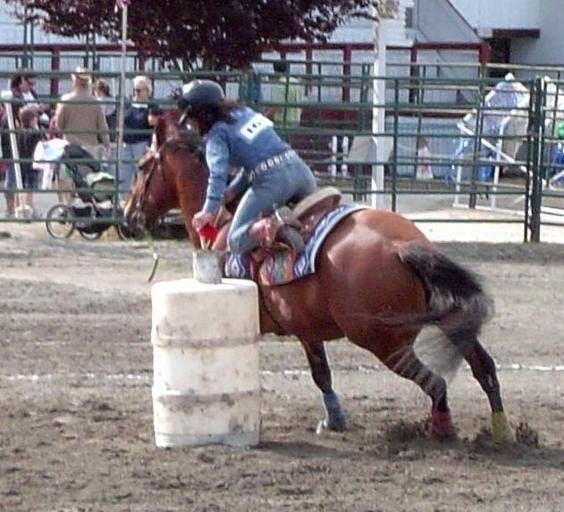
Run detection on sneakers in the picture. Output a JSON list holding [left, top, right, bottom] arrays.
[[59, 212, 67, 224]]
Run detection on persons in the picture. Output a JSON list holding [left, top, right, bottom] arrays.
[[172, 78, 321, 258], [0, 66, 167, 226]]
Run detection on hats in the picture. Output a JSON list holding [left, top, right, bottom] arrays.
[[72, 67, 91, 81], [133, 76, 149, 87]]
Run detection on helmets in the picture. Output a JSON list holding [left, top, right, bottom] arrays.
[[178, 79, 225, 127]]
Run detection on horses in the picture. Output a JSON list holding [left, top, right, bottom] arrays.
[[120, 101, 514, 444]]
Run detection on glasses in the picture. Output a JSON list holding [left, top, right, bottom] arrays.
[[25, 79, 37, 85]]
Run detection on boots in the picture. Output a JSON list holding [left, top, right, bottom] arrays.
[[252, 206, 305, 253]]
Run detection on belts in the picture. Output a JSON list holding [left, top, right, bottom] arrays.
[[247, 149, 294, 181]]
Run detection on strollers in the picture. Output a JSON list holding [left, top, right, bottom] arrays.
[[45, 144, 146, 243]]
